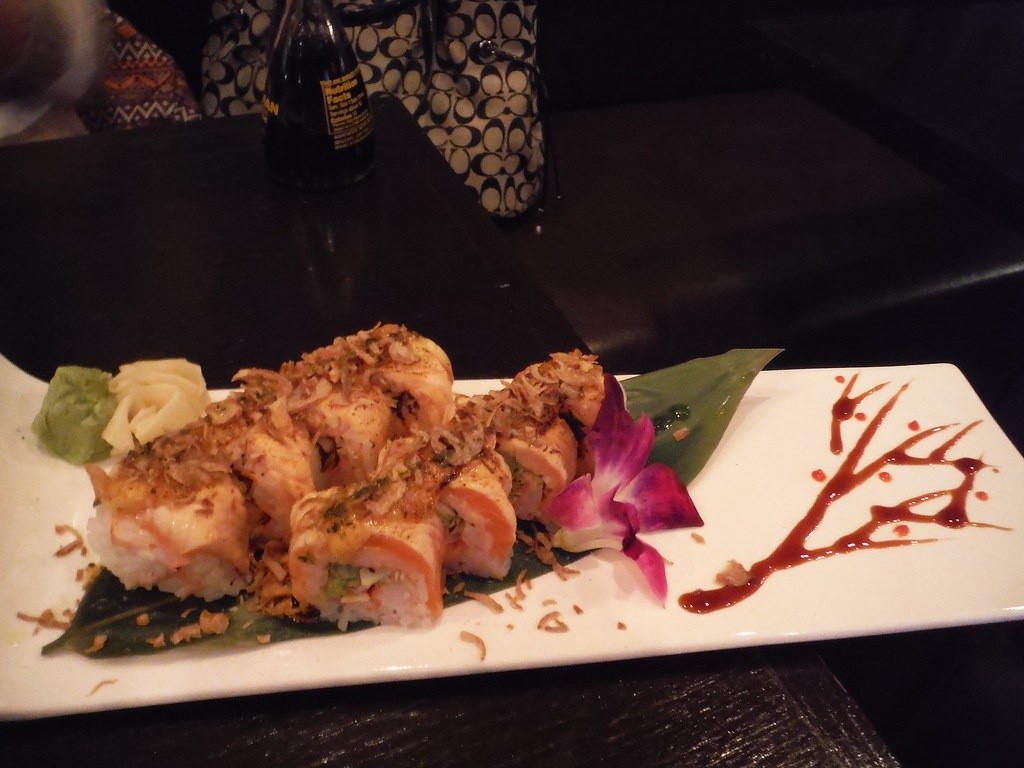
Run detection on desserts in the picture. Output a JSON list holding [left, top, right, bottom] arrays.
[[88, 323, 605, 630]]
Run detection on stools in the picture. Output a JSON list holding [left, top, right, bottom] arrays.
[[497, 0, 1023, 370]]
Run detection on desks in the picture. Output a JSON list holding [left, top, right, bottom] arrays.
[[745, 9, 1023, 202]]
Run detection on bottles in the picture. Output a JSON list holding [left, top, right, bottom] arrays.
[[265, 0, 378, 189]]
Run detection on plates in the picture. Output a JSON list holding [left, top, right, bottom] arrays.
[[0, 351, 1024, 721]]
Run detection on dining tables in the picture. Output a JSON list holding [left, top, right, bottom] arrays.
[[0, 90, 906, 768]]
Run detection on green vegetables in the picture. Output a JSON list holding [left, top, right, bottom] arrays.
[[31, 364, 110, 465]]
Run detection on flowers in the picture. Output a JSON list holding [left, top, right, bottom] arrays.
[[545, 368, 705, 611]]
